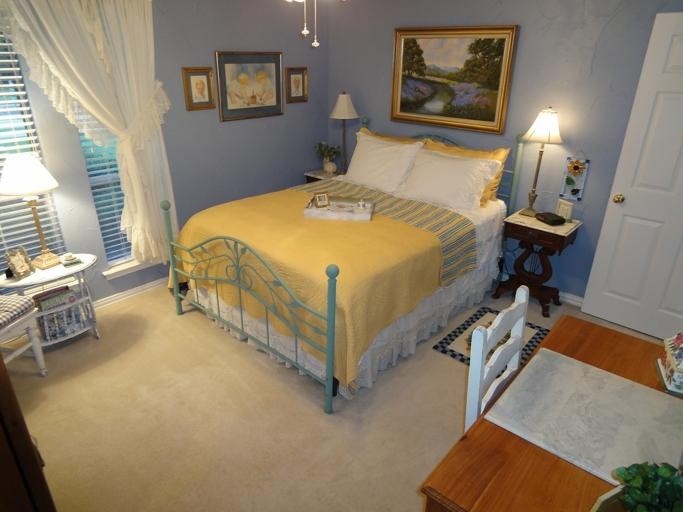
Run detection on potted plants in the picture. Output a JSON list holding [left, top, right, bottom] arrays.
[[592, 461, 683, 510], [314, 140, 345, 175]]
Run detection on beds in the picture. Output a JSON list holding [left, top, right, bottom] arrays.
[[157, 115, 526, 414]]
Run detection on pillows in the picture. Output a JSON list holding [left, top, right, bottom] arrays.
[[337, 129, 421, 199], [396, 148, 501, 217]]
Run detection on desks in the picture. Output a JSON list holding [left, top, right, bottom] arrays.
[[0, 251, 99, 350], [417, 315, 683, 511], [492, 207, 582, 317]]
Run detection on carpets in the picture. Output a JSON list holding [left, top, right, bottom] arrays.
[[431, 306, 551, 379]]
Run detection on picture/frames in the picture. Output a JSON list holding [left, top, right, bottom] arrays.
[[213, 51, 286, 122], [181, 66, 215, 112], [286, 67, 307, 106], [391, 23, 518, 134]]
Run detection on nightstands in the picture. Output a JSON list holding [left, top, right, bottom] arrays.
[[303, 167, 334, 181]]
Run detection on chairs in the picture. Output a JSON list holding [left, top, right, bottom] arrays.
[[0, 287, 50, 381], [464, 284, 531, 430]]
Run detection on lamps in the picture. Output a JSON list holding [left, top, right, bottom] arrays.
[[0, 149, 69, 269], [518, 103, 562, 217], [326, 93, 360, 174], [282, 1, 328, 48]]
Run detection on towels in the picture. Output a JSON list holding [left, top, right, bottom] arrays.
[[486, 347, 683, 489]]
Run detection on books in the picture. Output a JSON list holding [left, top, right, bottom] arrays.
[[30, 285, 81, 339]]
